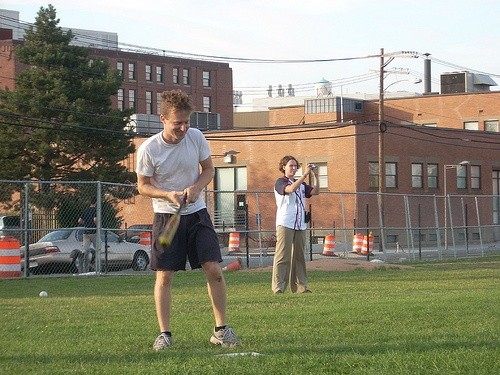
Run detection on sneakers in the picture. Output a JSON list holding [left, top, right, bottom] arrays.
[[152, 331, 173, 352], [90, 263, 96, 271]]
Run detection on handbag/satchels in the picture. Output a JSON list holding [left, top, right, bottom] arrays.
[[210, 325, 242, 349]]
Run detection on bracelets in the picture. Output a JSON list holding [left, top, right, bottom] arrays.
[[302, 174, 307, 178]]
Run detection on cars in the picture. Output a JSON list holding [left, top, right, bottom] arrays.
[[119, 224, 152, 240], [0, 215, 21, 237], [19, 226, 151, 275]]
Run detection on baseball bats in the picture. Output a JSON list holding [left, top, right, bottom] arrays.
[[157, 193, 187, 247]]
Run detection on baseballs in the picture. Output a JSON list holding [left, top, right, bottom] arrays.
[[39, 291, 48, 297]]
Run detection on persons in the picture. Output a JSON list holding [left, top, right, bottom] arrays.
[[135, 88, 244, 351], [77, 197, 98, 273], [271, 155, 320, 294]]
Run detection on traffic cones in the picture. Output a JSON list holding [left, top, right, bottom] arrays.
[[221, 257, 244, 272]]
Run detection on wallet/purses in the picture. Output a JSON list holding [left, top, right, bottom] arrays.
[[305, 211, 312, 224]]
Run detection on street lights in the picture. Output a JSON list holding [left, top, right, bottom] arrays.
[[444, 161, 472, 250], [378, 78, 423, 253], [204, 150, 241, 209]]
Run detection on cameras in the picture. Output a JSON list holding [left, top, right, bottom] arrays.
[[309, 163, 317, 170]]
